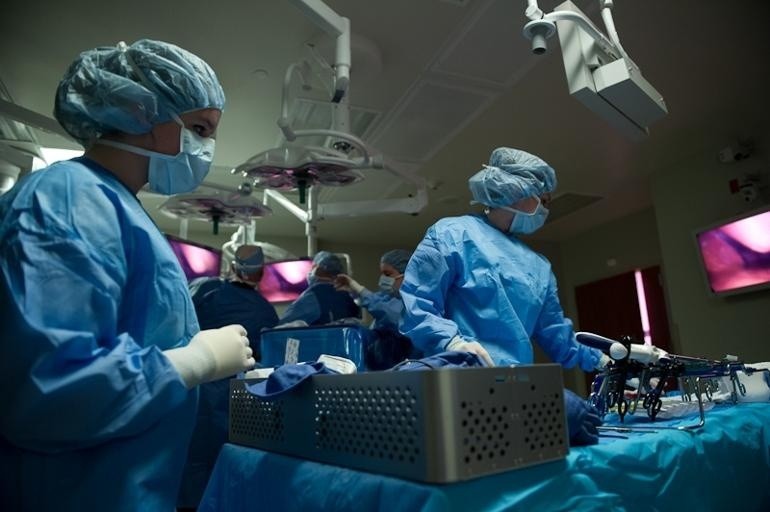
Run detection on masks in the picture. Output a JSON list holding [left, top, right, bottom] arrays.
[[494, 195, 550, 236], [96, 114, 219, 195], [378, 274, 405, 290]]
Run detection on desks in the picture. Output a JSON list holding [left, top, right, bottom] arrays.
[[217, 391, 770, 511]]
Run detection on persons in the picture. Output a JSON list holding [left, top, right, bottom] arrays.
[[333, 249, 415, 367], [397, 145, 613, 372], [191, 245, 278, 459], [276, 251, 360, 326], [0, 39, 258, 512]]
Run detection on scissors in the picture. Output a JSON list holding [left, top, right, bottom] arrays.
[[588, 370, 747, 424]]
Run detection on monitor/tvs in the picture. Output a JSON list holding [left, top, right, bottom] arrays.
[[161, 231, 223, 282], [690, 203, 770, 299], [257, 256, 314, 303]]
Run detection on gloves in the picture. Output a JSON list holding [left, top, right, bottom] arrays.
[[447, 335, 495, 369], [160, 323, 255, 390], [331, 273, 369, 297]]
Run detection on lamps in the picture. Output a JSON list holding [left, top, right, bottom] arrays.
[[155, 142, 366, 236]]
